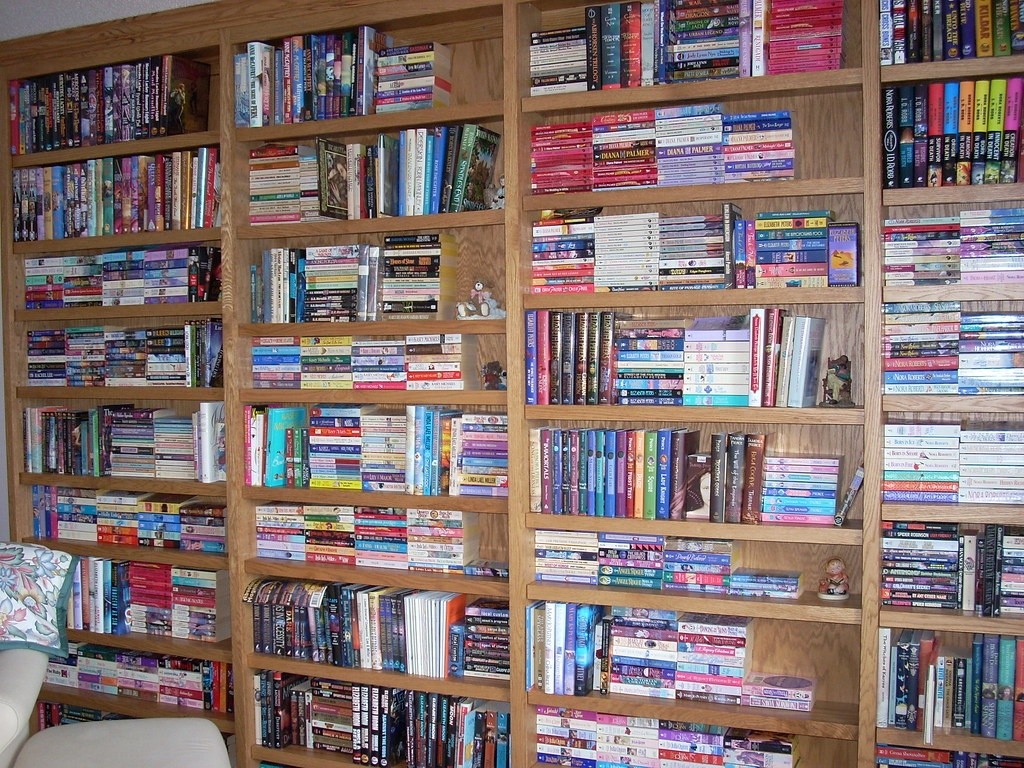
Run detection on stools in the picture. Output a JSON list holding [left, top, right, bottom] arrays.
[[14, 718, 232, 768]]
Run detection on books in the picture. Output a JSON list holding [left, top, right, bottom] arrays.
[[10, 0, 1024, 768]]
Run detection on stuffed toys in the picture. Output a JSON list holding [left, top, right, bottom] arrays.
[[479, 360, 507, 391], [457, 277, 506, 319]]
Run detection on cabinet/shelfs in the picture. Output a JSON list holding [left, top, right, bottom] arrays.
[[0, 0, 1024, 768]]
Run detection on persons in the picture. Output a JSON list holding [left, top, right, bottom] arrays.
[[828, 355, 851, 404], [823, 558, 850, 594]]
